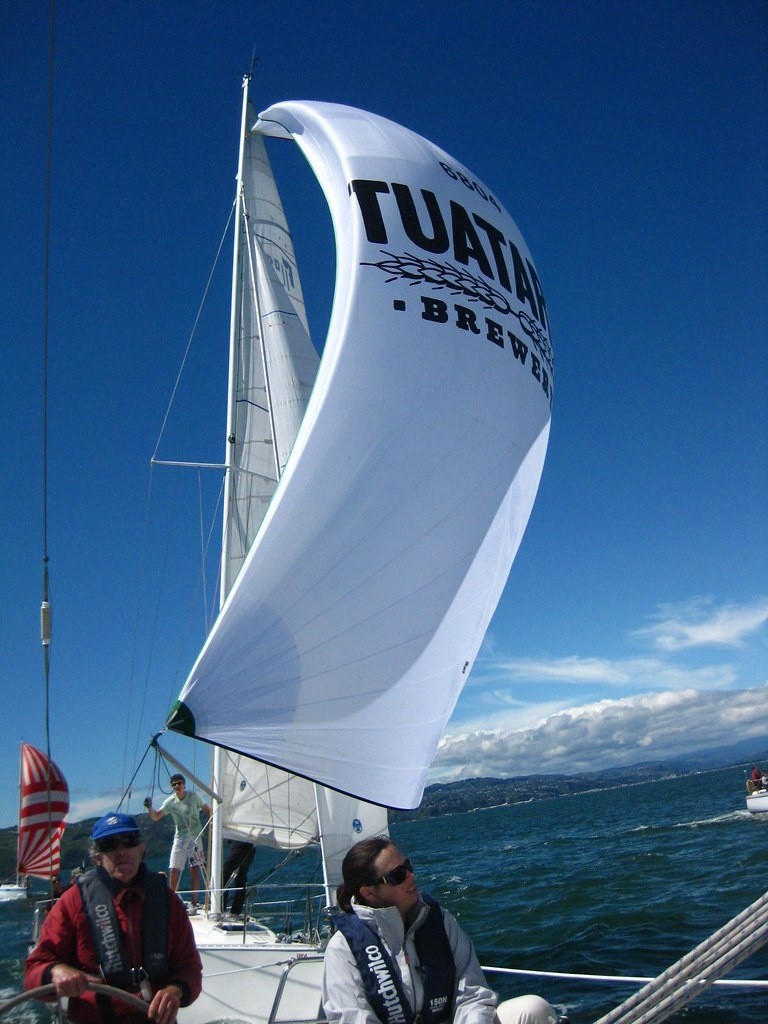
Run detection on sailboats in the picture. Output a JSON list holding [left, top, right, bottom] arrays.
[[1, 731, 72, 904]]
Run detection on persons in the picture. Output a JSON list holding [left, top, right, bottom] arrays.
[[44, 866, 85, 918], [751, 765, 768, 792], [144, 773, 211, 915], [25, 812, 203, 1024], [322, 836, 557, 1024]]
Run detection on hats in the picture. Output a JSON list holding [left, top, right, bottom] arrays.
[[763, 769, 768, 773], [752, 764, 758, 768], [72, 867, 83, 877], [90, 812, 141, 841]]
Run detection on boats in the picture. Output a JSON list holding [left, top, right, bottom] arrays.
[[743, 760, 768, 815]]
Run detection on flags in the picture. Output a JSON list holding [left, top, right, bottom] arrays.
[[19, 741, 70, 882]]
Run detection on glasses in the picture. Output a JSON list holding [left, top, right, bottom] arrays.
[[172, 781, 183, 786], [95, 835, 139, 852], [367, 858, 414, 887], [53, 878, 61, 882]]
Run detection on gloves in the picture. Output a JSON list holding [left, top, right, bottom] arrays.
[[143, 797, 153, 809]]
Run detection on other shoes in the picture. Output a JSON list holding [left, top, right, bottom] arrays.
[[188, 903, 198, 917], [225, 913, 239, 918]]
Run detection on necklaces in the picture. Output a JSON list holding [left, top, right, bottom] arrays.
[[222, 839, 256, 918]]
